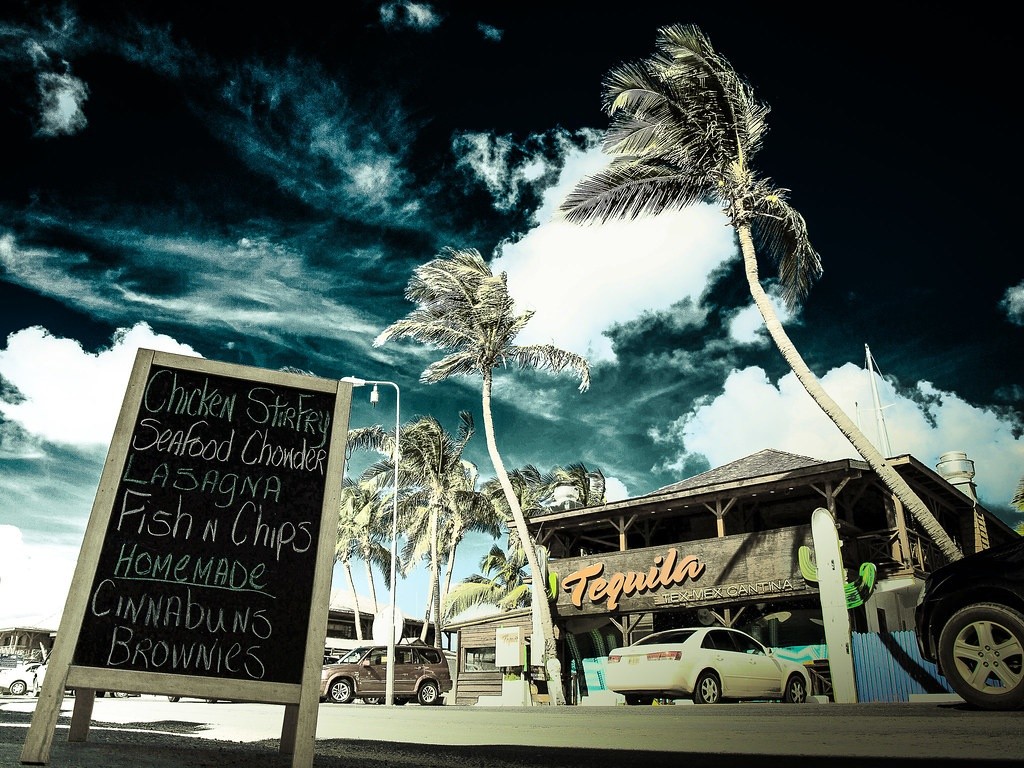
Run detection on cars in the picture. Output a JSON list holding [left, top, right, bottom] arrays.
[[33, 649, 106, 698], [606, 627, 811, 704], [314, 656, 382, 704], [914, 536, 1024, 711], [0, 663, 43, 695]]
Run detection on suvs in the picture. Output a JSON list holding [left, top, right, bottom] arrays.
[[319, 646, 454, 706]]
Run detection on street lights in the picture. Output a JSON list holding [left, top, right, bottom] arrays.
[[338, 375, 405, 707]]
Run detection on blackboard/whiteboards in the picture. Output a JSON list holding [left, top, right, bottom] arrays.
[[64, 347, 353, 705]]
